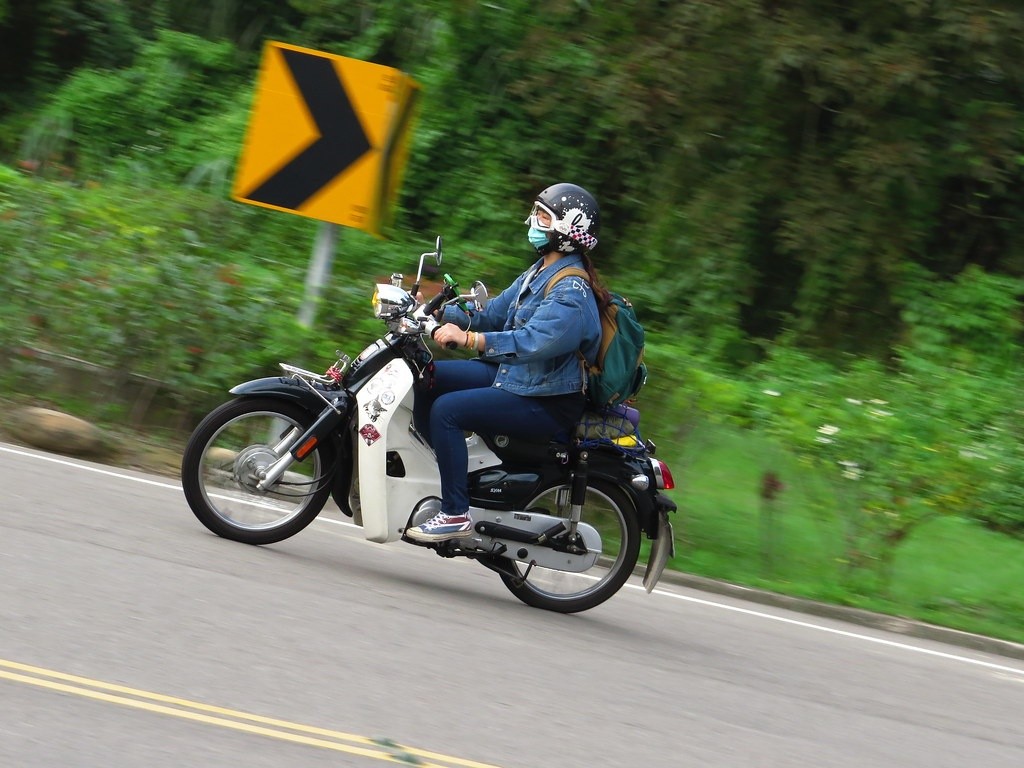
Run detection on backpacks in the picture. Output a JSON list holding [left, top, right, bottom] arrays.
[[544, 266, 648, 414]]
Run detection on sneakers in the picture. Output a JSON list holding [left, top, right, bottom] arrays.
[[406, 511, 475, 544]]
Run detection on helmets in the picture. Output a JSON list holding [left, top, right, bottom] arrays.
[[524, 182, 601, 252]]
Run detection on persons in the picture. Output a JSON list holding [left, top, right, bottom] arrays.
[[406, 183, 603, 543]]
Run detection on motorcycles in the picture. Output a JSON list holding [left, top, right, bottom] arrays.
[[179, 231, 684, 613]]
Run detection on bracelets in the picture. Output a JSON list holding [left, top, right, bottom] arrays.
[[464, 331, 479, 351]]
